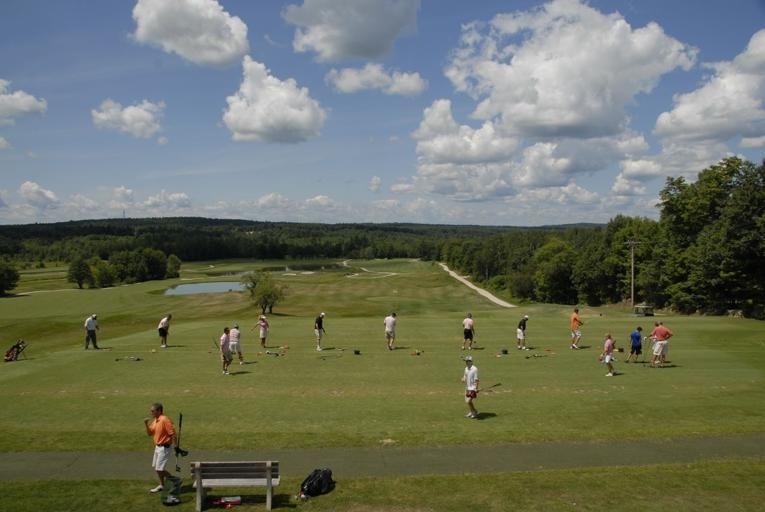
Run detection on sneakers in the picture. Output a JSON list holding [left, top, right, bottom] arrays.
[[467, 412, 472, 417], [472, 411, 477, 419], [605, 373, 613, 377], [151, 485, 162, 492]]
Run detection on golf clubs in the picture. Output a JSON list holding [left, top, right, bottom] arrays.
[[525, 334, 532, 347], [477, 382, 502, 398], [643, 334, 655, 365], [321, 329, 334, 348], [176, 410, 185, 468]]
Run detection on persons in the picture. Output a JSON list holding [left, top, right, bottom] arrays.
[[143, 402, 179, 494], [462, 355, 480, 418], [158, 314, 172, 348], [462, 314, 475, 349], [625, 326, 643, 363], [85, 314, 100, 350], [516, 315, 528, 350], [600, 333, 616, 377], [229, 324, 244, 365], [571, 308, 583, 349], [384, 313, 397, 350], [315, 312, 325, 351], [645, 321, 673, 367], [220, 327, 233, 374], [252, 315, 269, 347]]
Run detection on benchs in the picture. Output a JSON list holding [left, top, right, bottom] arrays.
[[190, 459, 281, 512]]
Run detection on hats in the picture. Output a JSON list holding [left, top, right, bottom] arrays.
[[464, 355, 473, 361]]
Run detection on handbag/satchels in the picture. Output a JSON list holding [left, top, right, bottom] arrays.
[[301, 467, 336, 497]]
